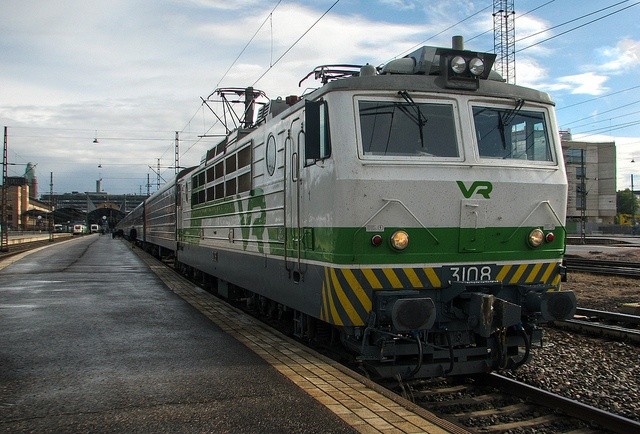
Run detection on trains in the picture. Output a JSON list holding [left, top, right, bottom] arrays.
[[72, 223, 89, 236], [112, 37, 577, 396], [90, 222, 102, 235]]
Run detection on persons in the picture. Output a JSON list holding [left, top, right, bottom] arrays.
[[129, 226, 137, 248]]
